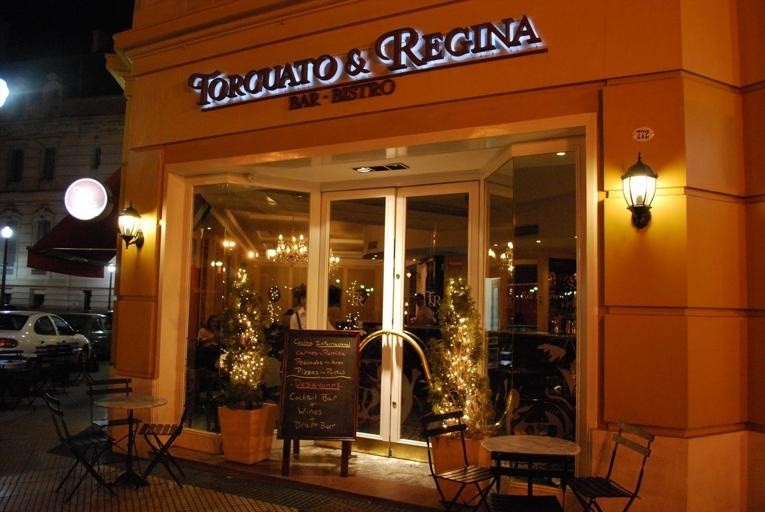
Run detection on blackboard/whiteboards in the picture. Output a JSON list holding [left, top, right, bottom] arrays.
[[277, 329, 361, 441]]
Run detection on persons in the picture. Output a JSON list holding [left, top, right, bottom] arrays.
[[287, 289, 339, 332], [409, 293, 437, 326], [197, 314, 222, 414], [282, 308, 295, 328]]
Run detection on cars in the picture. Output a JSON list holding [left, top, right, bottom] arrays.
[[1, 309, 112, 373]]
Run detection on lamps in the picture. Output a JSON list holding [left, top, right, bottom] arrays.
[[117, 200, 144, 250], [621, 151, 658, 229]]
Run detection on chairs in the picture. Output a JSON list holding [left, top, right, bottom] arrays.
[[421, 410, 656, 512], [42, 377, 196, 502]]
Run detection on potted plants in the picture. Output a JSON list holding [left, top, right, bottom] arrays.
[[214, 265, 278, 465], [423, 279, 494, 504]]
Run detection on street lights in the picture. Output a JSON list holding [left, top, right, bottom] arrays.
[[0, 226, 13, 310]]
